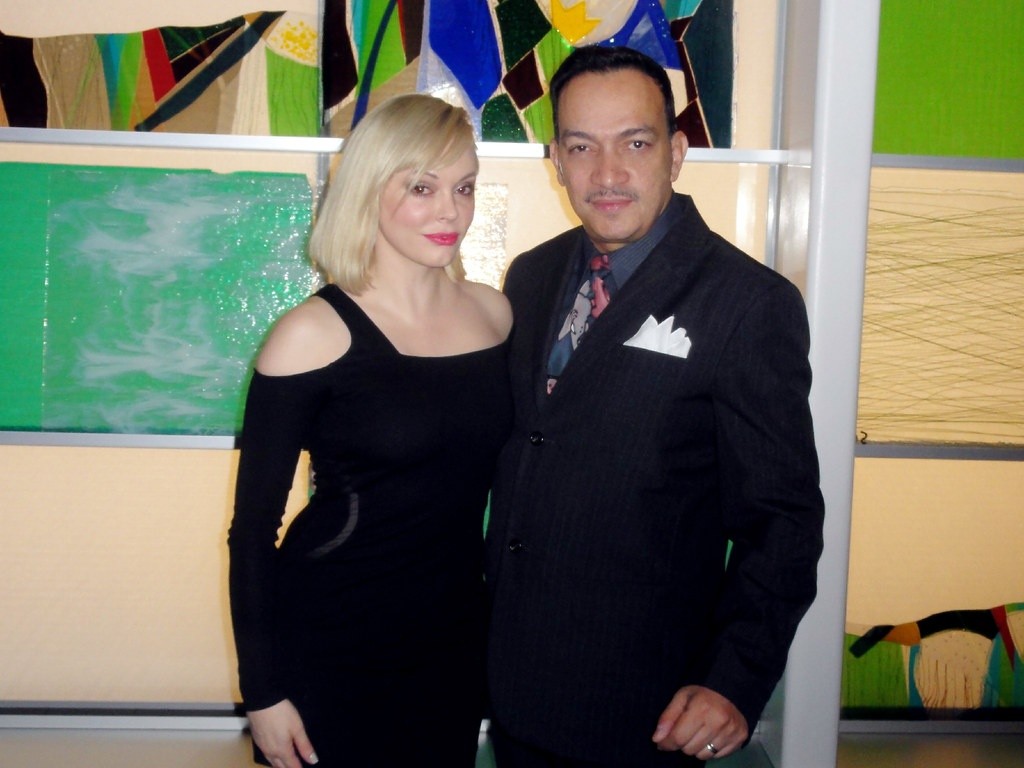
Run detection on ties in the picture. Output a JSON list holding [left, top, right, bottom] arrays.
[[547, 255, 612, 395]]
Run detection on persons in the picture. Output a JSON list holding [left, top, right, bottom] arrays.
[[225, 94, 514, 768], [483, 46, 826, 768]]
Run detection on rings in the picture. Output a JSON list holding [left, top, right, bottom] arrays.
[[708, 743, 719, 753]]
